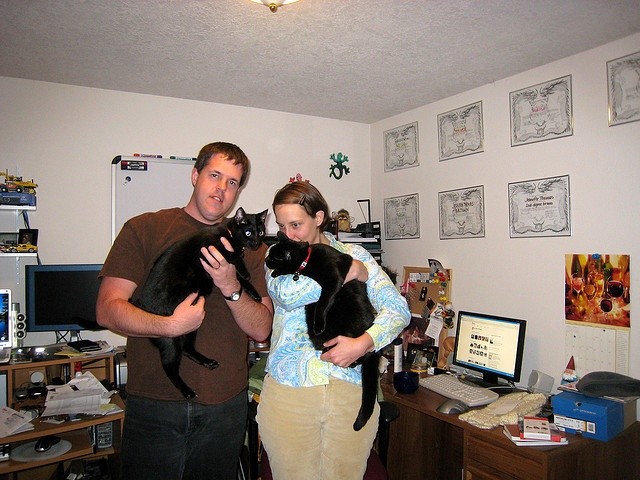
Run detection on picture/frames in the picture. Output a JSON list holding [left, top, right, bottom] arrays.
[[405, 342, 439, 367], [402, 266, 450, 316]]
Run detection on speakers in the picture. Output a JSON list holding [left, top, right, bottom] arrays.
[[528, 370, 555, 393], [445, 351, 466, 376]]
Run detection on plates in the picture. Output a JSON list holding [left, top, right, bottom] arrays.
[[11, 440, 74, 462]]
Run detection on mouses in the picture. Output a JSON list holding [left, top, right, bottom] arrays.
[[436, 398, 470, 414], [35, 435, 62, 452]]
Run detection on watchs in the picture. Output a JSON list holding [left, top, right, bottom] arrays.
[[223, 284, 243, 302]]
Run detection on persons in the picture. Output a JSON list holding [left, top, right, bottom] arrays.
[[95, 141, 274, 480], [256, 180, 412, 480]]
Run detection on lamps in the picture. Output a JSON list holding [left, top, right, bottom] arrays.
[[252, 0, 298, 12]]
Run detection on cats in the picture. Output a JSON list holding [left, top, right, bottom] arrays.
[[264, 230, 383, 432], [71, 206, 269, 400]]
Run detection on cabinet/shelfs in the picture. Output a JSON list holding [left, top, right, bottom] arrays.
[[0, 345, 127, 480], [0, 202, 38, 256], [380, 365, 640, 480]]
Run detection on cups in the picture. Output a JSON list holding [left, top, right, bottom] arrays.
[[393, 371, 419, 394]]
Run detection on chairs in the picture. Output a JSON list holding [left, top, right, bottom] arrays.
[[235, 400, 400, 480]]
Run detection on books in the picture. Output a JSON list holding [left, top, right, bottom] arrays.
[[53, 339, 114, 360], [502, 416, 569, 447]]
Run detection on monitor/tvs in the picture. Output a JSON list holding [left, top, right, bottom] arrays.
[[25, 263, 110, 341], [452, 311, 527, 394]]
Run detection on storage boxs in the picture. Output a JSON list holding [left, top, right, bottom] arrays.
[[550, 381, 639, 443]]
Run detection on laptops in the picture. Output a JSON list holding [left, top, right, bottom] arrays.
[[0, 288, 12, 366]]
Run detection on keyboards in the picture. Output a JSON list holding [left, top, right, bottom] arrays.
[[0, 444, 10, 462], [420, 373, 501, 409]]
